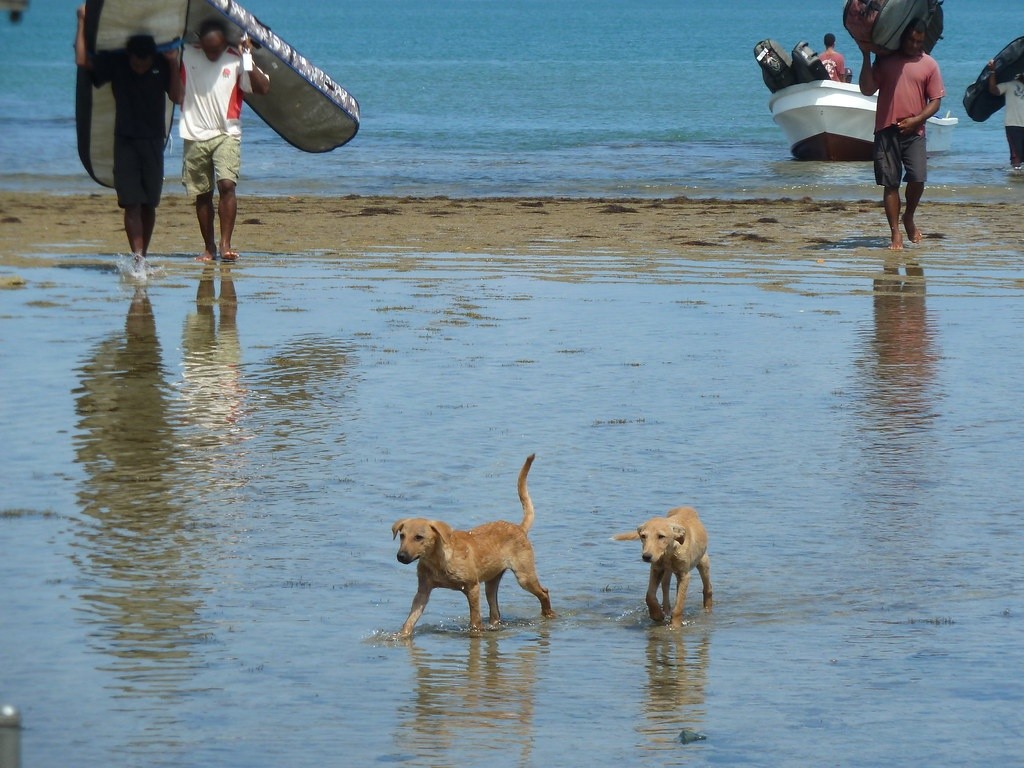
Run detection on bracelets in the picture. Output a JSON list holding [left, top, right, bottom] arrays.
[[991, 71, 995, 73]]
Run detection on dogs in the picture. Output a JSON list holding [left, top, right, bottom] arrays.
[[391, 452, 556, 638], [612, 506, 713, 629]]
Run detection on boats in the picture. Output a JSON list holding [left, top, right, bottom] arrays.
[[770, 79, 959, 161]]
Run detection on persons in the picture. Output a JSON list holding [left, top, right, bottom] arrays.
[[988, 59, 1024, 170], [74, 5, 181, 259], [175, 18, 270, 262], [859, 18, 946, 250], [817, 33, 845, 82]]
